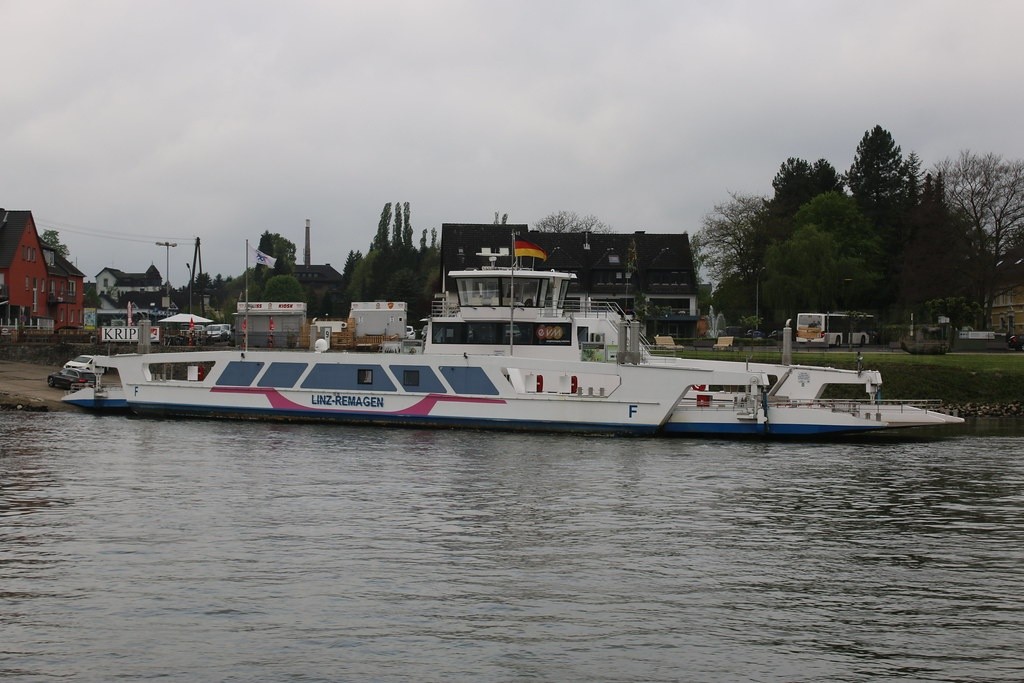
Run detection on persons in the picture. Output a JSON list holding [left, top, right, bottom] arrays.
[[807, 320, 816, 328], [816, 319, 821, 328]]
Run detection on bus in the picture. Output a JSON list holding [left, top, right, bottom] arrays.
[[796, 312, 877, 349]]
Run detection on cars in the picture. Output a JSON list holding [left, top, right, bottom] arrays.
[[63, 355, 109, 375], [746, 329, 765, 338], [222, 324, 232, 337], [407, 326, 417, 339], [195, 324, 207, 331], [207, 324, 224, 337], [48, 368, 96, 390], [768, 330, 783, 341]]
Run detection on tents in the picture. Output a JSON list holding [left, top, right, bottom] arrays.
[[158, 313, 214, 335]]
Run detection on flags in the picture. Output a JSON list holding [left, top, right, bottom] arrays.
[[248, 243, 277, 268], [513, 232, 547, 261]]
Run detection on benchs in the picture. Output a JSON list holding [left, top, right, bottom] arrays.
[[654, 337, 684, 352], [354, 336, 383, 352], [713, 336, 733, 351]]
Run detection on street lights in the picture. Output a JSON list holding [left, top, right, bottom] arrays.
[[186, 263, 192, 314], [756, 267, 766, 330], [156, 241, 178, 316]]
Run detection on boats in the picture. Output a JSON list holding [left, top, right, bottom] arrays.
[[62, 230, 966, 443]]
